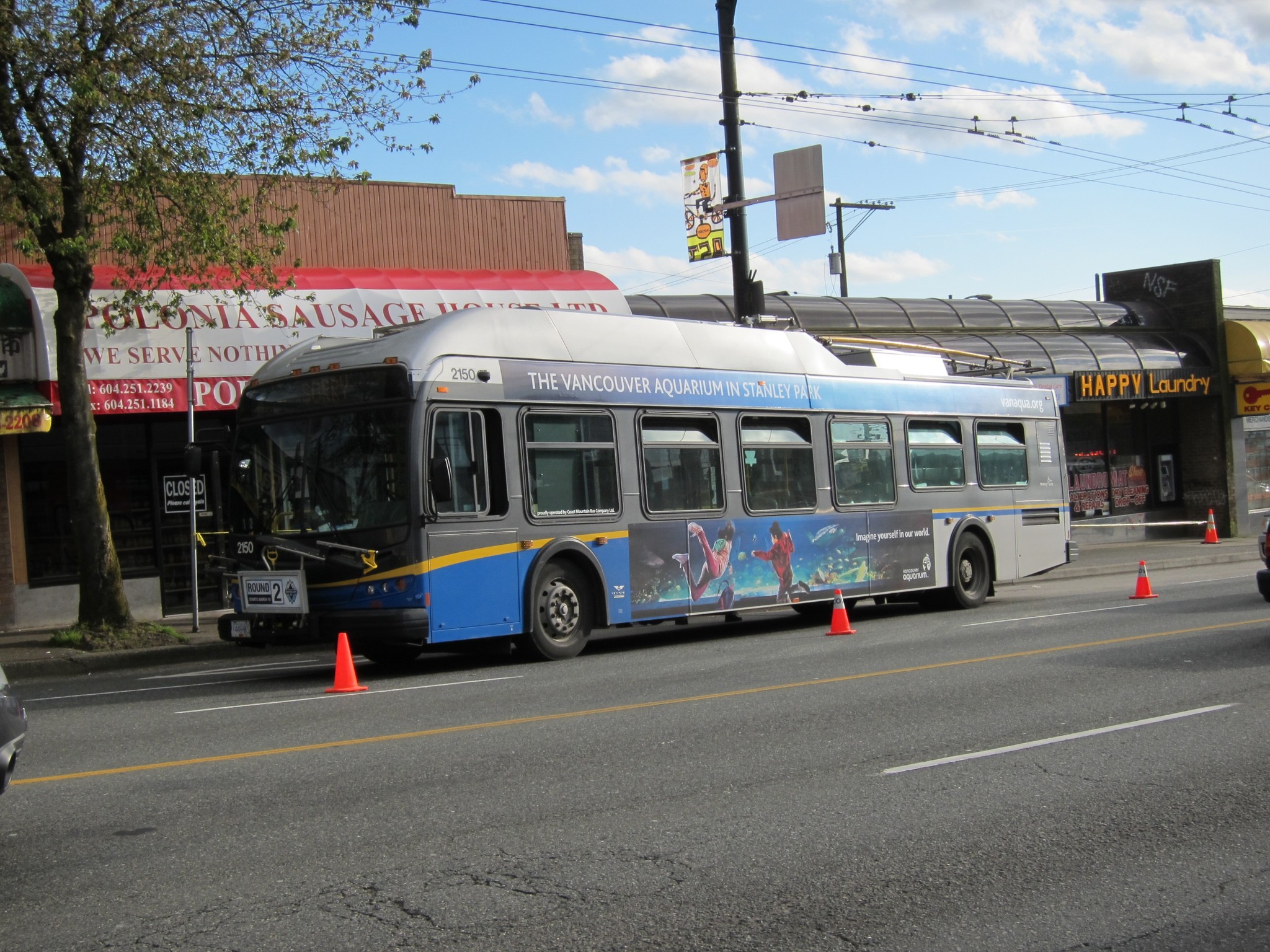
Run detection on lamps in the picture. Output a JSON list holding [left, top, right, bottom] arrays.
[[1129, 401, 1166, 410]]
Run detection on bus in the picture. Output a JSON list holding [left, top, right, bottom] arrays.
[[223, 311, 1072, 660]]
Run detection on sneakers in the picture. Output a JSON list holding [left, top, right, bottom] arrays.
[[687, 522, 703, 537], [786, 593, 793, 603], [672, 553, 689, 568], [798, 581, 810, 594]]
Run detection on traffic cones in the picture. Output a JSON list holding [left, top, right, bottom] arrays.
[[825, 589, 856, 636], [1129, 559, 1160, 599], [1200, 510, 1221, 544], [325, 631, 369, 695]]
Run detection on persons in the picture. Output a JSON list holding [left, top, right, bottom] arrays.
[[752, 522, 811, 604], [671, 521, 736, 602]]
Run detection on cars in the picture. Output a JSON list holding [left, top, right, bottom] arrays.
[[0, 663, 28, 794], [1255, 518, 1270, 601]]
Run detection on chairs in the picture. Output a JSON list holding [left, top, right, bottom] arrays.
[[653, 452, 1029, 510]]
